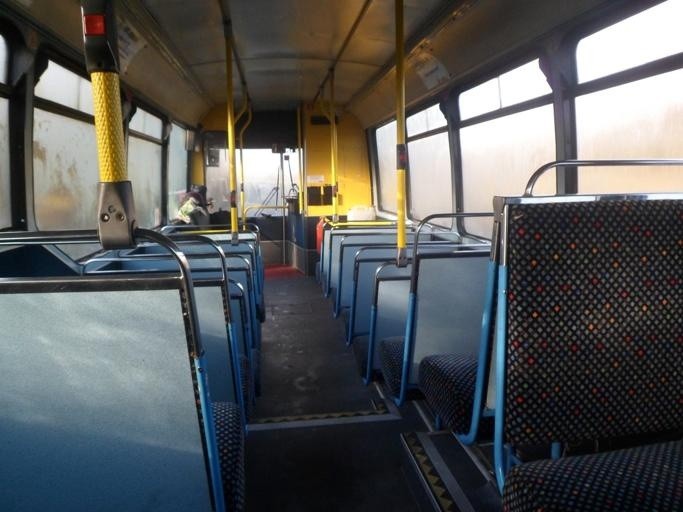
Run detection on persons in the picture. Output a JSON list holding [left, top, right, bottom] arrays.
[[173, 184, 214, 226]]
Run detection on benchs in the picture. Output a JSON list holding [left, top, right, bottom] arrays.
[[421, 168, 683, 510], [313, 221, 490, 409], [2, 221, 265, 510]]
